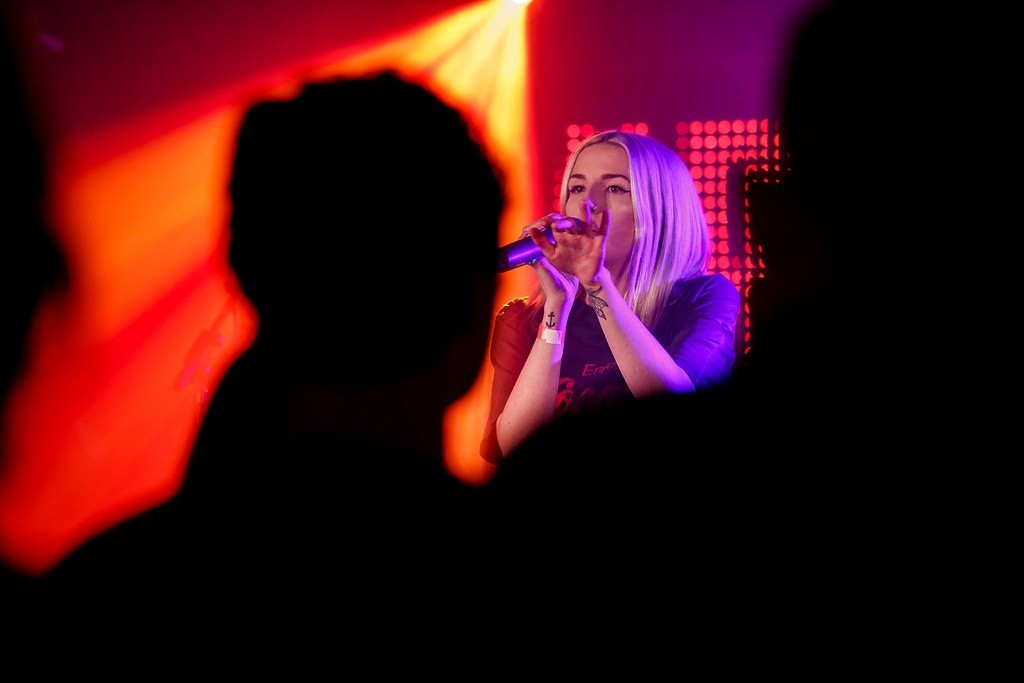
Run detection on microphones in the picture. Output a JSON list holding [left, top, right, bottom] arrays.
[[497, 217, 583, 273]]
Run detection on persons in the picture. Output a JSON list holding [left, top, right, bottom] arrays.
[[0, 0, 1024, 683], [479, 128, 742, 464]]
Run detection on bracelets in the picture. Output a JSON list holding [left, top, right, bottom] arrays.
[[537, 323, 565, 344]]
